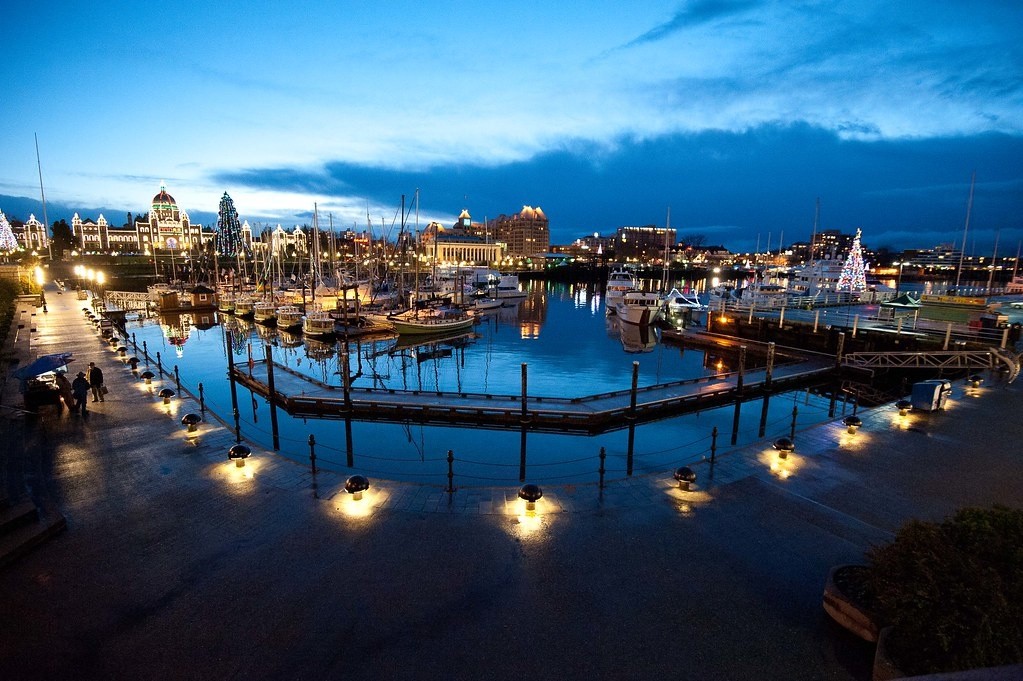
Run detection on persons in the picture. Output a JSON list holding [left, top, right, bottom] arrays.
[[86, 362, 105, 402], [71, 372, 90, 415], [54, 372, 74, 411]]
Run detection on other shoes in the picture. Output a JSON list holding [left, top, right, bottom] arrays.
[[100, 400, 104, 402], [92, 399, 98, 402]]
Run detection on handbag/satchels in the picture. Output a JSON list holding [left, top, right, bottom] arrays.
[[101, 385, 108, 394]]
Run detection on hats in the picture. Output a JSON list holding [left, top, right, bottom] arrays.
[[76, 372, 86, 376], [88, 362, 94, 366]]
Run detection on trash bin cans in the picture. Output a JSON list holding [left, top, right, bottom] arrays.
[[910, 379, 953, 413]]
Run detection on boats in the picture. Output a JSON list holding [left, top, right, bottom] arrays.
[[605, 230, 893, 325]]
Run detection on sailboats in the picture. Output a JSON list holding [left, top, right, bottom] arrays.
[[212, 188, 528, 337]]
